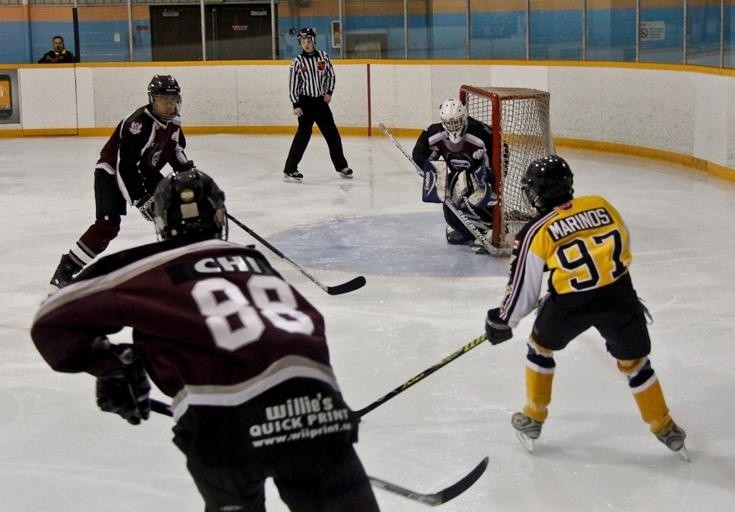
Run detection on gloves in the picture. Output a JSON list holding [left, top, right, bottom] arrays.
[[485, 308, 510, 345], [93, 343, 152, 426]]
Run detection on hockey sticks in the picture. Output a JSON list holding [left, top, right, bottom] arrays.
[[379, 122, 513, 257], [225, 212, 366, 296], [149, 399, 488, 507]]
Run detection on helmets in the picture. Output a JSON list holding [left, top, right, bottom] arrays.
[[152, 167, 227, 247], [437, 97, 470, 142], [296, 26, 316, 47], [146, 73, 183, 118], [520, 155, 576, 208]]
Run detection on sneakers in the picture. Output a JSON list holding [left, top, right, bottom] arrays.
[[658, 424, 687, 451], [286, 170, 303, 179], [43, 267, 73, 286], [338, 168, 352, 176], [509, 413, 544, 440]]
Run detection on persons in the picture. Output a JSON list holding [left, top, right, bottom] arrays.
[[38, 36, 78, 63], [49, 74, 199, 291], [30, 171, 381, 512], [282, 28, 354, 182], [412, 100, 510, 255], [486, 154, 694, 461]]
